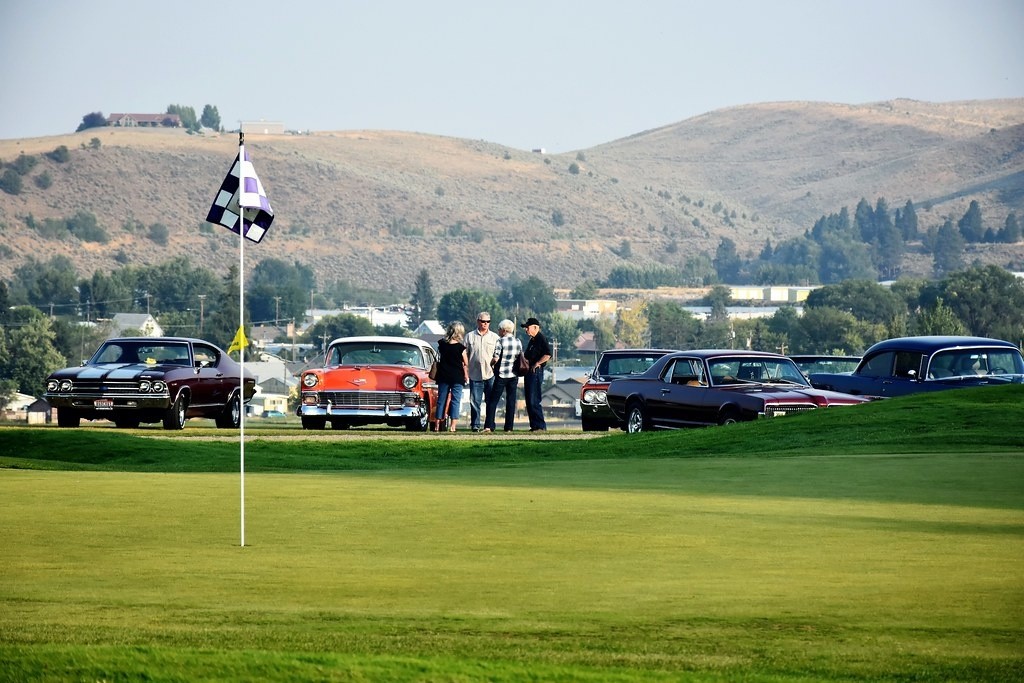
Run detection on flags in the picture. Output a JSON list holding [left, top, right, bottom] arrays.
[[205, 146, 275, 243]]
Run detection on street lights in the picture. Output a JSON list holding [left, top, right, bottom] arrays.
[[185, 307, 205, 337]]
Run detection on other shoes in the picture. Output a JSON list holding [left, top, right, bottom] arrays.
[[473, 428, 479, 432], [530, 428, 546, 433], [435, 428, 439, 432], [483, 428, 491, 432], [506, 430, 512, 433], [450, 427, 456, 432], [491, 427, 495, 431]]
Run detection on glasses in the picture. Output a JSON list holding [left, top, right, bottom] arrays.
[[479, 319, 490, 323]]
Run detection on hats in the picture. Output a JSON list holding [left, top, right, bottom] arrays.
[[521, 317, 539, 327]]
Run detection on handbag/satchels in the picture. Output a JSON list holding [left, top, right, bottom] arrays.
[[429, 349, 440, 379], [517, 351, 530, 375]]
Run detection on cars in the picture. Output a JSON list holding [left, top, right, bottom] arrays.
[[577, 347, 702, 432], [768, 353, 873, 385], [603, 347, 873, 435], [806, 334, 1024, 401], [294, 335, 452, 432], [43, 335, 258, 430]]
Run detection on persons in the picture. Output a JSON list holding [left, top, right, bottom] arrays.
[[520, 317, 552, 432], [463, 312, 500, 431], [480, 319, 523, 432], [434, 321, 469, 432]]
